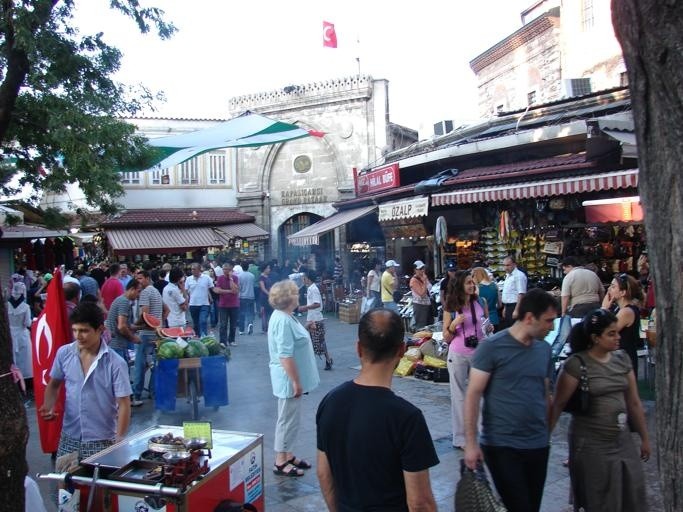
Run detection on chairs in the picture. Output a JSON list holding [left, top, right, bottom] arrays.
[[331, 282, 347, 317]]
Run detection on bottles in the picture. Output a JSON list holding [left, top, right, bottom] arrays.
[[480, 313, 485, 323], [648, 314, 655, 331]]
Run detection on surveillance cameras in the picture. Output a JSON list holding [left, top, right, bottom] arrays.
[[266, 193, 271, 199]]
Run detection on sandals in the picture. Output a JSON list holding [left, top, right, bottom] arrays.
[[290, 455, 312, 471], [272, 461, 304, 478]]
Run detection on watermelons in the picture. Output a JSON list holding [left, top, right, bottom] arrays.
[[141, 311, 161, 328], [158, 337, 221, 359], [156, 327, 194, 337]]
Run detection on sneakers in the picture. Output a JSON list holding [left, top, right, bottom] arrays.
[[220, 342, 226, 349], [247, 323, 254, 335], [239, 331, 244, 335], [229, 342, 238, 347], [131, 400, 143, 407], [325, 357, 334, 370]]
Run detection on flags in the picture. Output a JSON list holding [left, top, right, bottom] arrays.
[[322, 20, 338, 48]]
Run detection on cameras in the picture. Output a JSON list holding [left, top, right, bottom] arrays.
[[465, 336, 479, 348]]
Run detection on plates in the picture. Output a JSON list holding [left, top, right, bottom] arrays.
[[181, 438, 207, 450], [162, 451, 190, 463]]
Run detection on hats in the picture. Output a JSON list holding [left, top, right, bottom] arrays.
[[444, 261, 457, 272], [412, 260, 426, 269], [385, 260, 401, 267]]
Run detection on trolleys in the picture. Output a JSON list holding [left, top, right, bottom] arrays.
[[32, 421, 271, 512], [146, 345, 228, 420]]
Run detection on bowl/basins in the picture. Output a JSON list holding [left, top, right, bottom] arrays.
[[139, 450, 163, 462], [147, 438, 185, 454]]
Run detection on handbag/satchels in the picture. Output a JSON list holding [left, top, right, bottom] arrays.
[[452, 457, 506, 512], [556, 355, 594, 418], [393, 290, 403, 302]]
